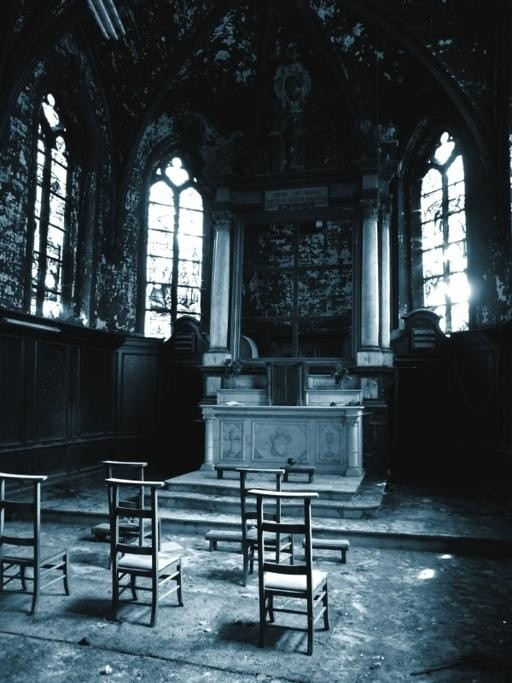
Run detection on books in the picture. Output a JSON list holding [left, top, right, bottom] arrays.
[[175, 332, 193, 351], [411, 327, 436, 350]]
